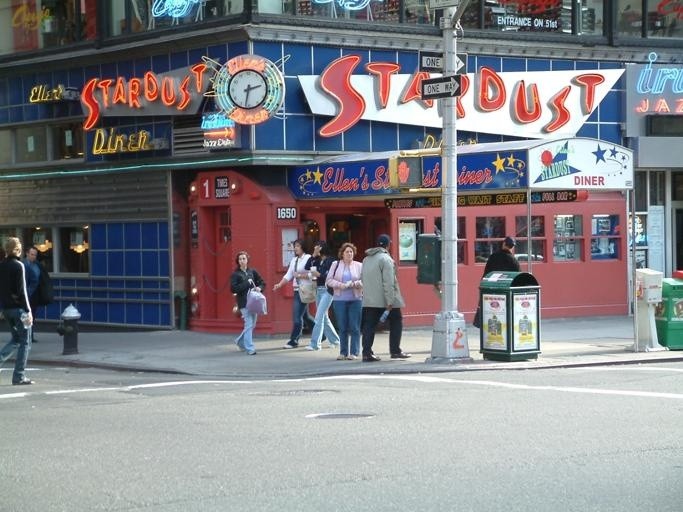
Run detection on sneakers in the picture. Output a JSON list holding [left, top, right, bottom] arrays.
[[345, 354, 359, 359], [283, 345, 294, 348], [337, 354, 348, 360], [304, 346, 314, 351], [12, 378, 34, 385]]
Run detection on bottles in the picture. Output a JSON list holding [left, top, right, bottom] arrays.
[[378, 309, 390, 324], [18, 309, 31, 330]]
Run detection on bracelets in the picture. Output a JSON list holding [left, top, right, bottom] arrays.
[[298, 273, 302, 279]]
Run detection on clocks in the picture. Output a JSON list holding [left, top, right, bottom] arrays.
[[200, 50, 292, 126]]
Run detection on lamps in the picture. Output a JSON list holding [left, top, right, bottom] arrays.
[[32, 229, 52, 253], [69, 225, 88, 254]]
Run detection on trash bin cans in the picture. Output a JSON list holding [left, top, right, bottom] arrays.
[[480, 271, 542, 361], [655, 278, 683, 350]]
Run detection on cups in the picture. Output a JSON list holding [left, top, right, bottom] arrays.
[[310, 266, 317, 279]]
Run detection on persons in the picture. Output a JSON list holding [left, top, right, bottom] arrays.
[[361, 233, 411, 362], [230, 251, 265, 355], [472, 237, 522, 353], [272, 239, 326, 349], [1, 236, 42, 386], [304, 238, 340, 350], [325, 241, 363, 359]]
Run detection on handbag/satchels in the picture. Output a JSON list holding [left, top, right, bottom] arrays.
[[327, 288, 334, 296], [298, 283, 316, 304], [246, 291, 268, 315]]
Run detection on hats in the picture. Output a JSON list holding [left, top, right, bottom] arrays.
[[376, 234, 391, 243]]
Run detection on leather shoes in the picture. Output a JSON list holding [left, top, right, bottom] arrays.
[[391, 352, 412, 358], [363, 354, 382, 361]]
